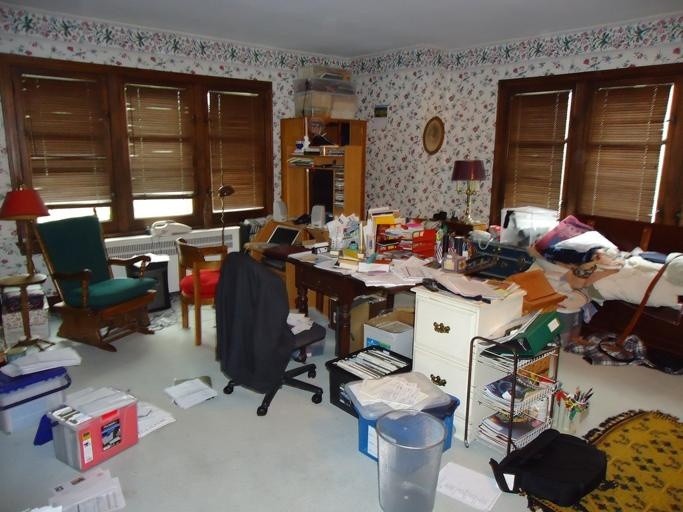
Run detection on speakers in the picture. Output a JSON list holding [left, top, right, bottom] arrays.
[[310, 205, 326, 228]]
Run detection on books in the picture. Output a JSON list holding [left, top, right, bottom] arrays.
[[334, 167, 344, 202], [0, 346, 134, 428], [302, 203, 560, 304], [478, 308, 561, 449], [287, 148, 344, 166], [377, 320, 413, 335], [332, 349, 408, 407]]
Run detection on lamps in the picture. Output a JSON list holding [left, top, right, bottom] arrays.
[[0, 184, 52, 276], [451, 158, 488, 223]]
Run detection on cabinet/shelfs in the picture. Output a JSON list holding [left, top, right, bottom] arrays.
[[279, 114, 369, 224], [411, 293, 524, 448]]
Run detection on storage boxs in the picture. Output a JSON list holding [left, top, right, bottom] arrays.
[[0, 362, 139, 474], [499, 204, 562, 252], [293, 63, 360, 120], [322, 305, 463, 477]]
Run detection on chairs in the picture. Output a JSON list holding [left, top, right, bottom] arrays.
[[175, 237, 330, 419], [35, 214, 157, 352]]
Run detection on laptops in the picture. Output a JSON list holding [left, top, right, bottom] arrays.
[[253, 224, 300, 251]]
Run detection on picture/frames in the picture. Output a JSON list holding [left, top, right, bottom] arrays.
[[422, 116, 444, 155]]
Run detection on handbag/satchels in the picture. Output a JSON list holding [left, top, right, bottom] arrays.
[[490, 429, 606, 507]]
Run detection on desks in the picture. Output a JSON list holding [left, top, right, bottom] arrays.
[[0, 272, 51, 356], [288, 242, 420, 356]]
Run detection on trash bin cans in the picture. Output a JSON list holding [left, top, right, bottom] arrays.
[[376, 410, 448, 512]]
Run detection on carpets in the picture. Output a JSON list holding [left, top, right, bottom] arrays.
[[531, 408, 683, 511]]
